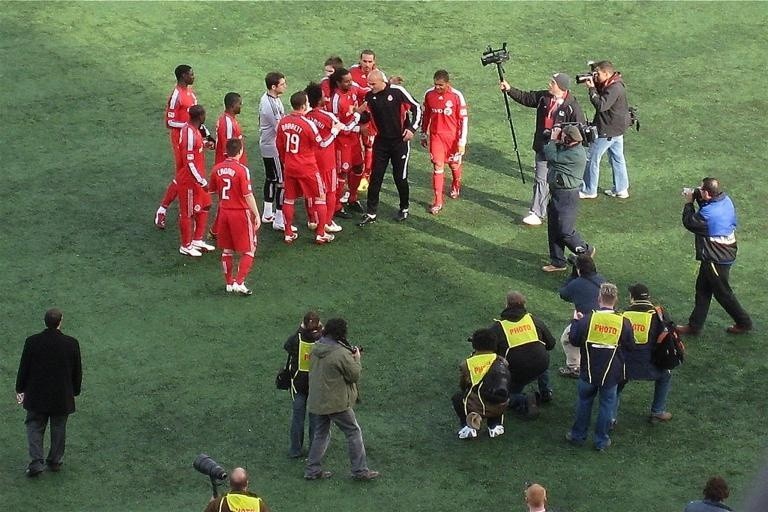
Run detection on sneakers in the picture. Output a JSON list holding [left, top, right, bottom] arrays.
[[289, 447, 381, 481], [488, 425, 504, 438], [226, 282, 253, 296], [579, 189, 630, 200], [429, 185, 462, 215], [178, 240, 216, 258], [396, 209, 409, 222], [648, 410, 672, 425], [542, 246, 597, 273], [591, 417, 619, 451], [537, 389, 553, 403], [26, 461, 62, 477], [565, 432, 585, 446], [558, 366, 580, 379], [522, 213, 545, 226], [261, 200, 378, 245], [524, 392, 539, 420], [457, 426, 478, 439], [154, 207, 167, 229]]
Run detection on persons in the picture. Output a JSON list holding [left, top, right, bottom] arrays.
[[304, 317, 380, 482], [419, 69, 468, 213], [498, 70, 585, 230], [671, 178, 753, 336], [156, 64, 216, 256], [683, 476, 735, 511], [17, 310, 82, 478], [556, 250, 607, 378], [287, 311, 325, 460], [449, 329, 513, 439], [206, 92, 261, 294], [202, 465, 268, 511], [491, 291, 557, 420], [541, 122, 595, 272], [257, 50, 421, 244], [578, 61, 634, 201], [564, 283, 635, 449], [617, 283, 681, 424], [524, 483, 548, 511]]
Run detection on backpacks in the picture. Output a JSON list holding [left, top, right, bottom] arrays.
[[650, 323, 685, 370]]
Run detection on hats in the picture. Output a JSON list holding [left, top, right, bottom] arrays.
[[562, 124, 583, 142], [552, 73, 570, 91]]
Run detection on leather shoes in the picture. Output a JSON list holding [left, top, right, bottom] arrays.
[[675, 324, 702, 334], [727, 323, 753, 334]]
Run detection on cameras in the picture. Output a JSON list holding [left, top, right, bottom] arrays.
[[466, 335, 474, 343], [352, 344, 363, 352], [682, 187, 700, 199], [567, 254, 577, 266], [575, 60, 599, 84]]
